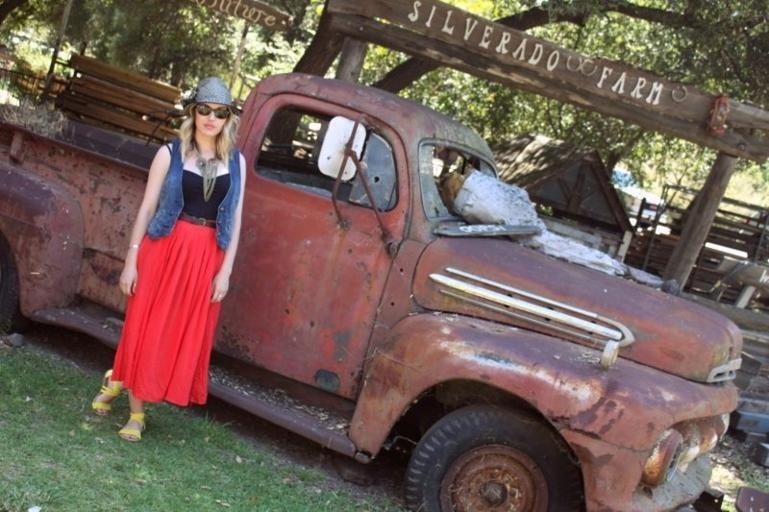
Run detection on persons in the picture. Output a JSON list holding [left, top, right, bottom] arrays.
[[90, 76, 248, 441]]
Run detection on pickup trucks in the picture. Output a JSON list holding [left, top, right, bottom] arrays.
[[0, 71, 743, 510]]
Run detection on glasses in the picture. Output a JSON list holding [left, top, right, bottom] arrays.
[[197, 104, 230, 119]]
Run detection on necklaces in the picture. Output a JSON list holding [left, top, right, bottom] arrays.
[[192, 140, 222, 203]]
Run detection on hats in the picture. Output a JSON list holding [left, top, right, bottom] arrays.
[[192, 76, 232, 105]]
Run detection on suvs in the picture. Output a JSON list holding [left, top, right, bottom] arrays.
[[515, 135, 662, 218]]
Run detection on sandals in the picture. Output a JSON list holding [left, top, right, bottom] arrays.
[[118, 412, 146, 442], [91, 369, 123, 417]]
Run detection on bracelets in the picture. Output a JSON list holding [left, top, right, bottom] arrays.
[[129, 244, 139, 250]]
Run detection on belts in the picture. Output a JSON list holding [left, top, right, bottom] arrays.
[[178, 211, 218, 227]]
